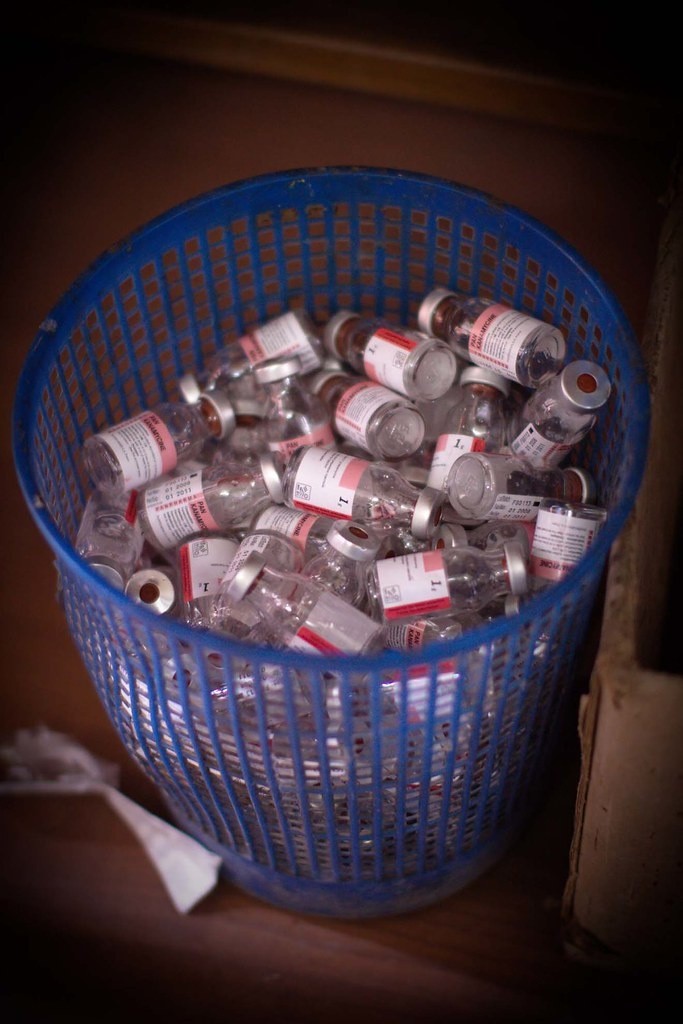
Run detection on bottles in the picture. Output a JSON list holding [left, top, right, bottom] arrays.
[[74, 287, 612, 876]]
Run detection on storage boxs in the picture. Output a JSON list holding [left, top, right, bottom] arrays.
[[556, 152, 683, 958]]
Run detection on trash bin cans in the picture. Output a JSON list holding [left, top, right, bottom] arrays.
[[10, 165, 649, 918]]
